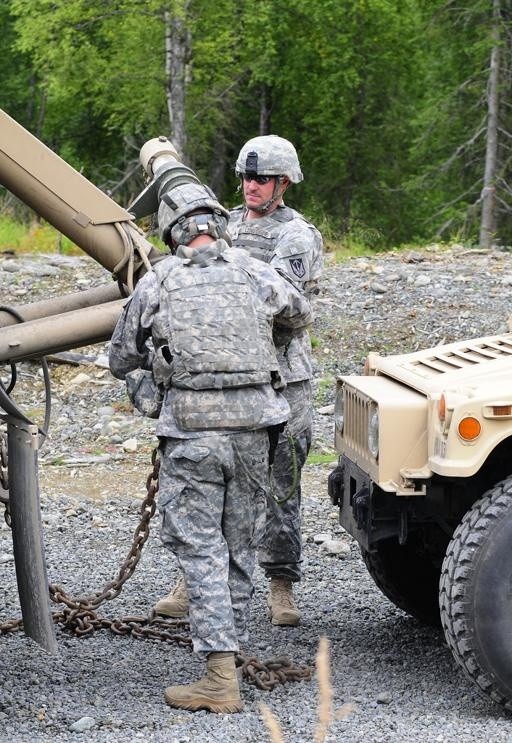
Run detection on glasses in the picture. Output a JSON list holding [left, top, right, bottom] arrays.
[[243, 172, 271, 184]]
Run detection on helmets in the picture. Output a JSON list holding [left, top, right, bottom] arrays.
[[235, 133, 305, 186], [159, 182, 229, 242]]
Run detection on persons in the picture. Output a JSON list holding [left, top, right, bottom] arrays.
[[109, 179, 315, 717], [153, 131, 321, 628]]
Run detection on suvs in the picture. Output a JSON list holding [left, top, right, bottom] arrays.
[[328, 322, 511, 722]]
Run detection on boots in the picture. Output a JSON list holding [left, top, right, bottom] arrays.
[[266, 575, 301, 627], [154, 575, 189, 617], [163, 652, 244, 714]]
